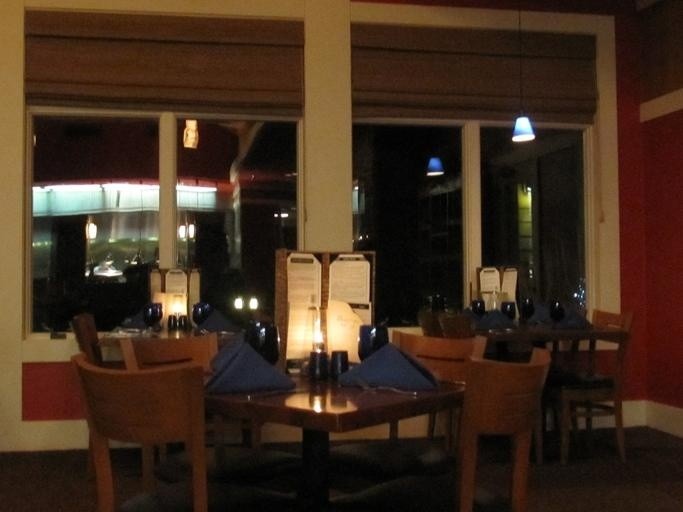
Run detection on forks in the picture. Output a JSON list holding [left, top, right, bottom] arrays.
[[355, 376, 419, 397]]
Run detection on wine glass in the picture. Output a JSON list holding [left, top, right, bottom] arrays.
[[145, 301, 214, 336]]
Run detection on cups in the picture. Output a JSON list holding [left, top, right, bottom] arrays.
[[472, 299, 567, 328], [356, 325, 391, 362], [309, 351, 350, 382], [244, 318, 281, 366]]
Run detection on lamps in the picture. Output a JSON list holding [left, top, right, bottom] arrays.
[[426, 158, 445, 177], [512, 0, 536, 143]]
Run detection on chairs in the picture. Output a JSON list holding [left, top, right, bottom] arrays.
[[418, 308, 472, 340], [120, 331, 220, 498], [75, 310, 105, 363], [72, 349, 210, 512], [556, 309, 633, 464], [456, 346, 551, 510], [392, 328, 487, 389]]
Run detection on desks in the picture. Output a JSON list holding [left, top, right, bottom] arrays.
[[206, 360, 456, 512], [467, 315, 627, 360]]
